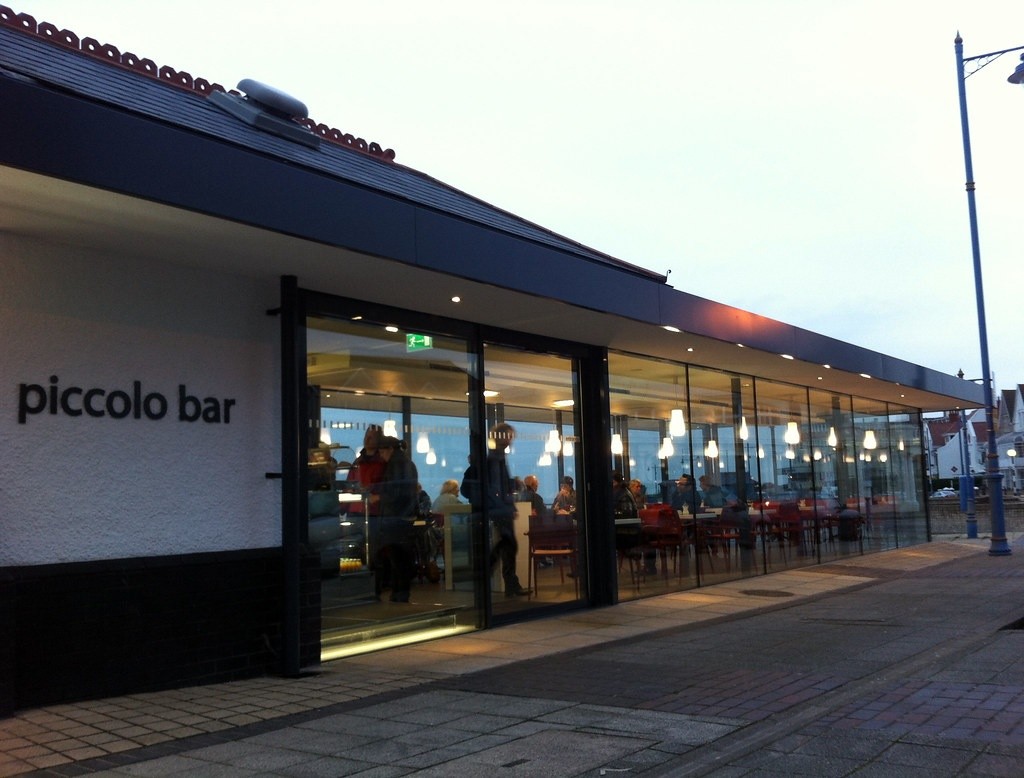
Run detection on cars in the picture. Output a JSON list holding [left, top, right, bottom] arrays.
[[873, 485, 982, 507]]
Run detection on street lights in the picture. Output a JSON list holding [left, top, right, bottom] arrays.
[[1006, 449, 1017, 496], [951, 29, 1024, 554]]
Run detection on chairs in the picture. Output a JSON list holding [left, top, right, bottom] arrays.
[[638, 495, 901, 583], [525, 514, 579, 598]]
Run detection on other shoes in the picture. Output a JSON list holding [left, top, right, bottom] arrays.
[[505, 589, 533, 598], [638, 567, 657, 576]]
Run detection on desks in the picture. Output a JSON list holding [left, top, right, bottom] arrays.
[[615, 518, 641, 524], [616, 513, 717, 579], [707, 508, 776, 565]]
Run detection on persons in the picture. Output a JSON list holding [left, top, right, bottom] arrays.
[[486, 422, 533, 598], [342, 422, 385, 568], [519, 476, 549, 569], [417, 484, 432, 519], [550, 476, 576, 521], [431, 479, 473, 569], [460, 454, 475, 505], [610, 470, 764, 577], [366, 436, 417, 603]]
